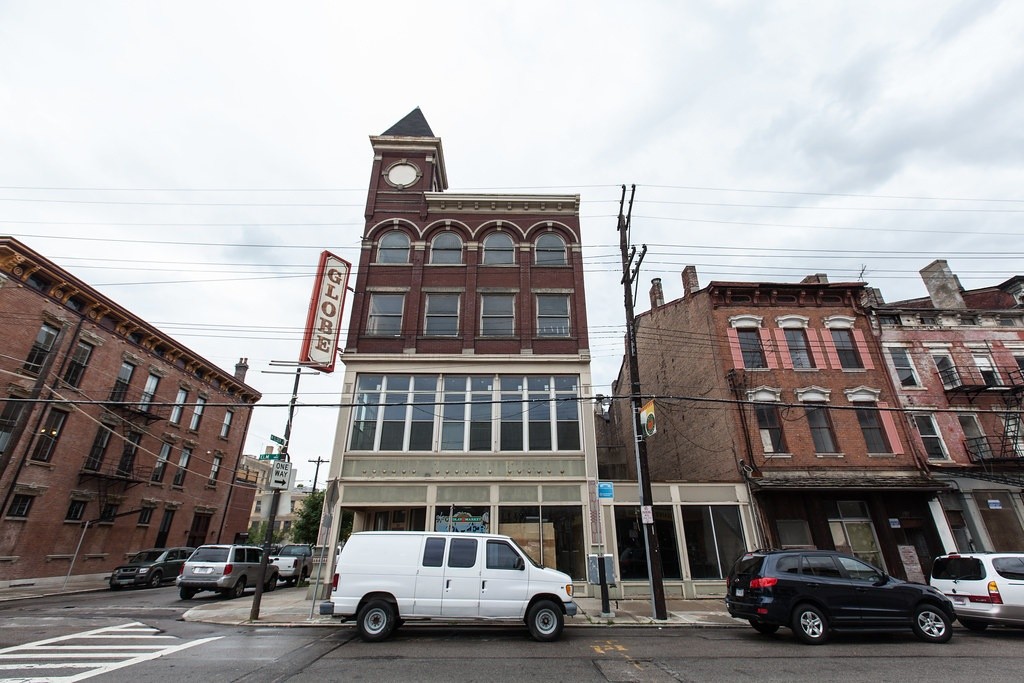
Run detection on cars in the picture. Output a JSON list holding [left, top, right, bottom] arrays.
[[109, 547, 195, 592], [928, 551, 1024, 635]]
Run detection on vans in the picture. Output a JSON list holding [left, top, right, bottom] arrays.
[[318, 530, 578, 643]]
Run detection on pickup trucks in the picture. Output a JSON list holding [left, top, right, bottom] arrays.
[[265, 543, 315, 584]]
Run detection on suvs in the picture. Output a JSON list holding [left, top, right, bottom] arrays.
[[176, 544, 280, 598], [724, 547, 959, 644]]
